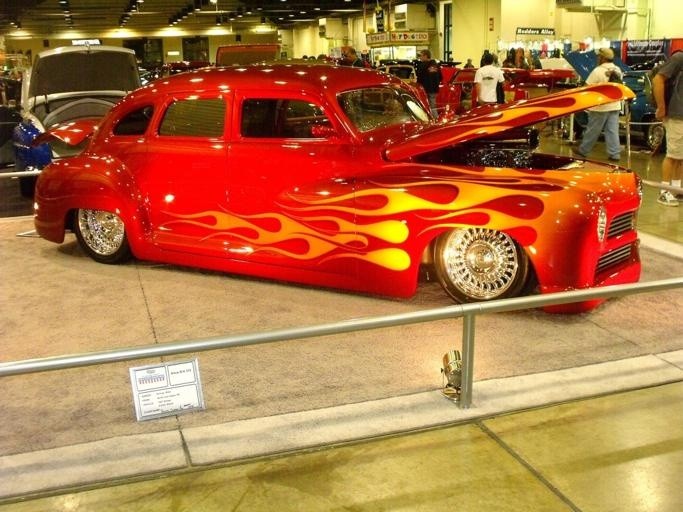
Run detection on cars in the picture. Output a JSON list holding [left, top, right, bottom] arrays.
[[34, 62, 642, 317], [5, 40, 674, 200]]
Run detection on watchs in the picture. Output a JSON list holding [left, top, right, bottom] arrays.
[[417, 50, 442, 120]]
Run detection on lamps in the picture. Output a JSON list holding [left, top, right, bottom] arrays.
[[4, 0, 267, 29]]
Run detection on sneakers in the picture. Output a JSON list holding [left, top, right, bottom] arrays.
[[608, 156, 619, 162], [656, 190, 679, 207], [570, 144, 586, 156]]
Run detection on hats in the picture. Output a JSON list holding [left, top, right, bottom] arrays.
[[598, 47, 615, 61]]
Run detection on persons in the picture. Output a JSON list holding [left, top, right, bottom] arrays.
[[342, 48, 364, 67], [650, 48, 682, 206], [569, 46, 622, 161], [479, 47, 548, 71], [472, 53, 505, 105]]
